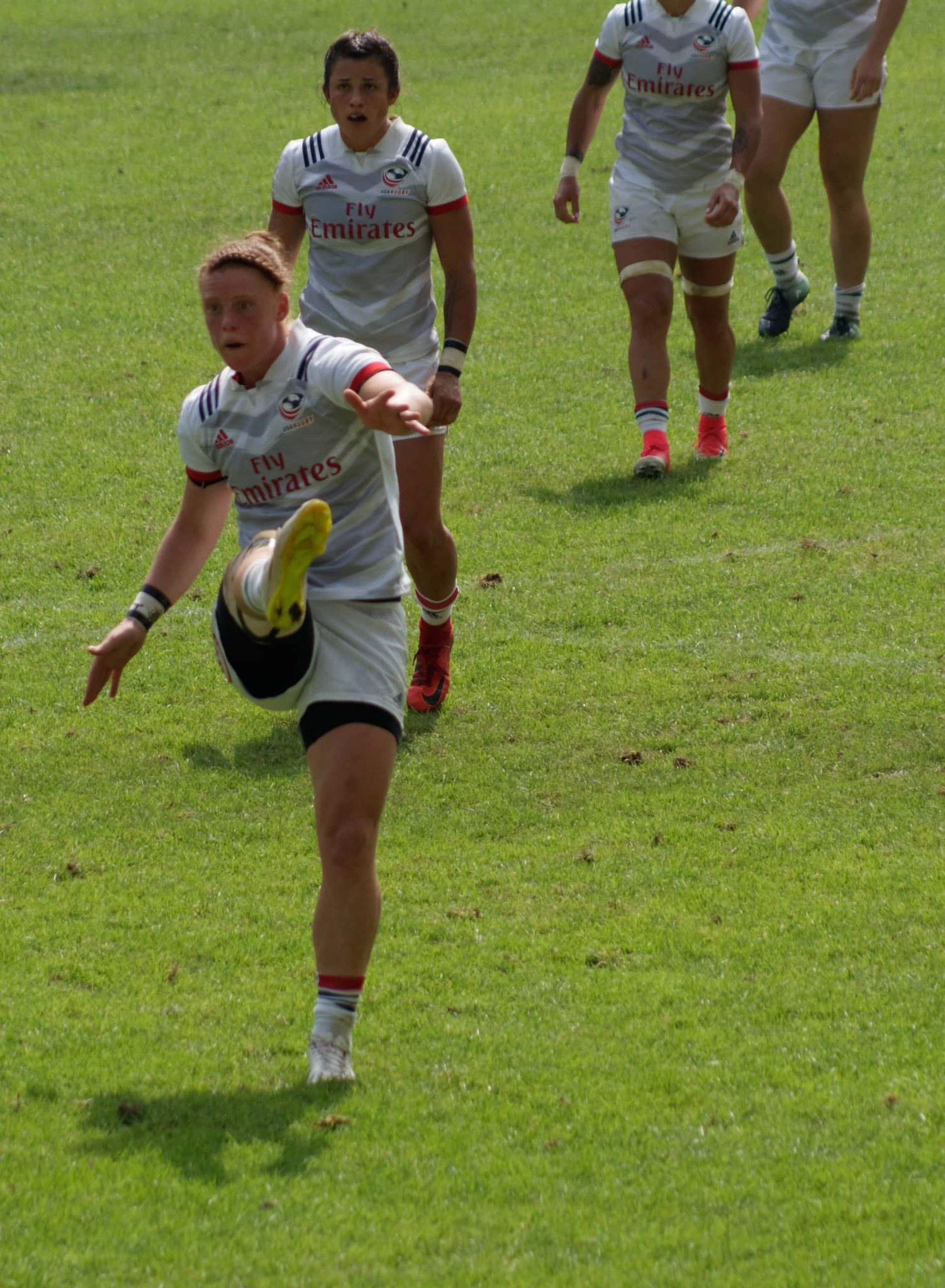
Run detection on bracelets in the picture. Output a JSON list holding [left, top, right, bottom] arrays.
[[559, 150, 584, 179], [722, 168, 745, 193], [125, 583, 171, 632], [437, 338, 468, 379]]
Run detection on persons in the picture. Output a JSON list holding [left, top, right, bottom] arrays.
[[268, 28, 477, 713], [83, 229, 434, 1091], [553, 0, 764, 477], [732, 0, 908, 346]]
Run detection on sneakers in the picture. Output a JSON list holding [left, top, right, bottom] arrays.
[[693, 424, 728, 461], [820, 315, 860, 342], [633, 444, 670, 476], [309, 1035, 355, 1083], [759, 275, 809, 338], [264, 498, 332, 629], [406, 629, 454, 712]]
